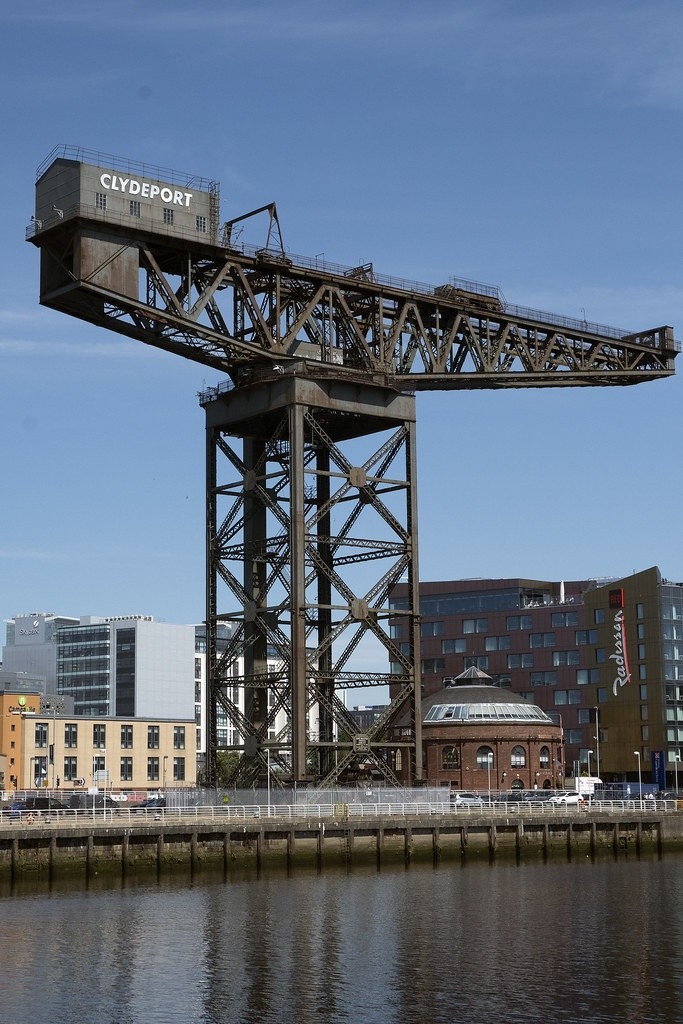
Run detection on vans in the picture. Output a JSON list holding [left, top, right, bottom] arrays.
[[653, 792, 675, 807], [619, 792, 656, 808], [70, 794, 120, 814]]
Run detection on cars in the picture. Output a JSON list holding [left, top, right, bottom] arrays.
[[450, 790, 484, 808], [3, 797, 76, 819], [498, 788, 584, 806], [130, 798, 166, 813]]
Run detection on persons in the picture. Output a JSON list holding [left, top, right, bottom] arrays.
[[644, 792, 648, 800], [649, 792, 654, 800], [158, 787, 161, 799]]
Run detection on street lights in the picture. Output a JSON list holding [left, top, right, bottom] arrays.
[[487, 752, 494, 807], [587, 749, 594, 808], [634, 751, 643, 809], [675, 755, 680, 795]]
